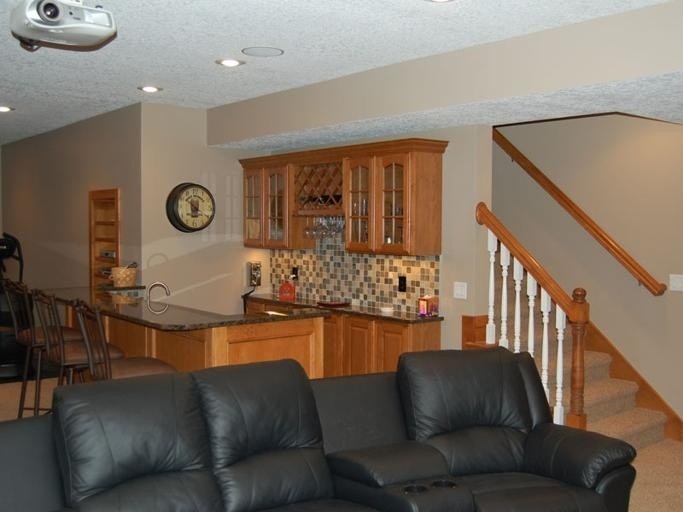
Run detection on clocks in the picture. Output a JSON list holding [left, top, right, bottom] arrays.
[[165, 181, 216, 233]]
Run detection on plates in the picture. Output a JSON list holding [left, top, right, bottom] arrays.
[[316, 302, 348, 306]]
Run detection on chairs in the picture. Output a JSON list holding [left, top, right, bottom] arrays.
[[31, 286, 119, 416], [68, 295, 178, 383], [0, 275, 84, 420]]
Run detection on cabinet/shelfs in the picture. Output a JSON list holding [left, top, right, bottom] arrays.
[[87, 187, 120, 287], [344, 139, 442, 257], [244, 298, 288, 314], [342, 311, 439, 373], [291, 154, 344, 217], [239, 155, 312, 250], [318, 311, 342, 378]]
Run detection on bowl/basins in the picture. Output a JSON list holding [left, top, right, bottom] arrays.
[[381, 307, 394, 314]]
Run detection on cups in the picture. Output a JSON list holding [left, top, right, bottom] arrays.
[[109, 267, 137, 288]]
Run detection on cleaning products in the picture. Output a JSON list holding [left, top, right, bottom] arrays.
[[279, 281, 295, 301]]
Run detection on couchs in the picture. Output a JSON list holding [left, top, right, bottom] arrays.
[[1, 347, 643, 512]]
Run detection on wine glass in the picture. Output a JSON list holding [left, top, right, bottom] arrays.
[[302, 215, 345, 240]]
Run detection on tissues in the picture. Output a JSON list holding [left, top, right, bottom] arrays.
[[418, 295, 438, 316]]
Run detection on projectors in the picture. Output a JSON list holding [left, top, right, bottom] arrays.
[[8, 1, 118, 53]]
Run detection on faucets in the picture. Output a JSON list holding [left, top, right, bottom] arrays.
[[146, 301, 169, 316], [146, 281, 170, 302]]
